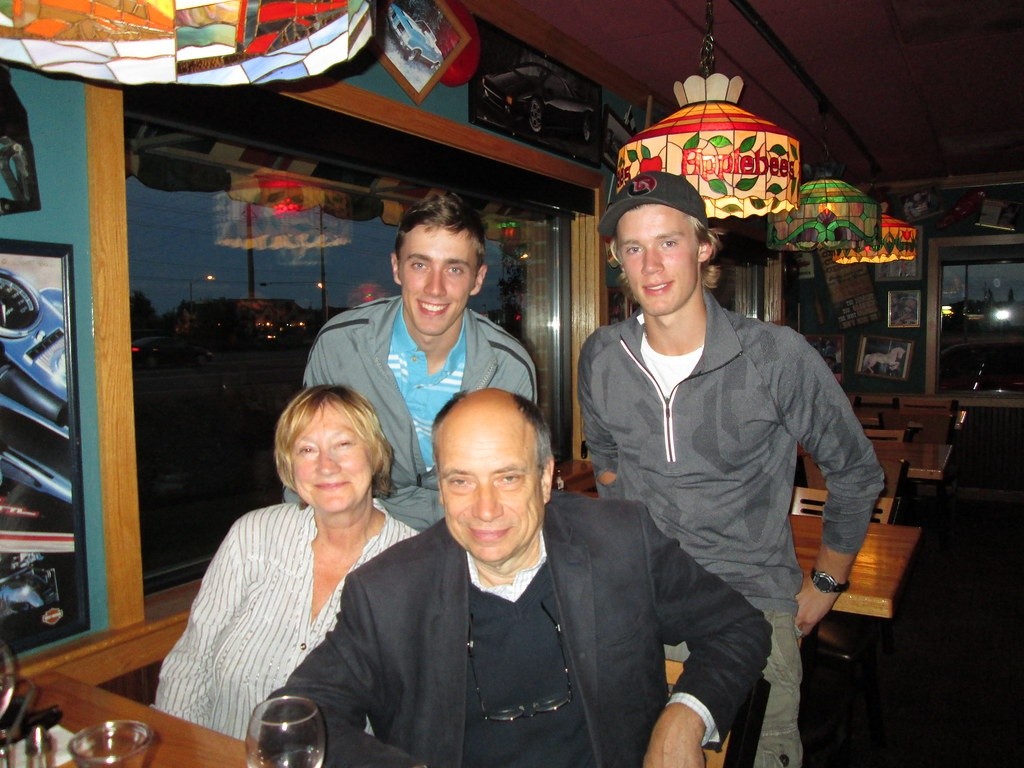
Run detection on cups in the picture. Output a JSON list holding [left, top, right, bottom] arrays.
[[245, 695, 327, 768], [66, 721, 154, 768]]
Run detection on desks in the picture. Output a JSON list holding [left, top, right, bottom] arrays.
[[0, 668, 275, 768], [871, 439, 955, 482]]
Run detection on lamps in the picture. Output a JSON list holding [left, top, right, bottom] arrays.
[[833, 167, 919, 266], [766, 102, 883, 257], [612, 2, 799, 220]]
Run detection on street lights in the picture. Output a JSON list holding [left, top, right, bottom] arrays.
[[189, 275, 215, 338], [309, 282, 324, 331]]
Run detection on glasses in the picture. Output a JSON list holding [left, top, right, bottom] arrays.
[[467, 601, 573, 721]]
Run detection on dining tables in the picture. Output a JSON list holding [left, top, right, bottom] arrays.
[[787, 514, 923, 767]]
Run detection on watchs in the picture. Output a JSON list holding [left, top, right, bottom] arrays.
[[811, 567, 850, 594]]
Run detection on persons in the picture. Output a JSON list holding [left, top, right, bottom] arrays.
[[279, 192, 540, 541], [577, 169, 886, 768], [260, 386, 775, 768], [148, 382, 422, 743]]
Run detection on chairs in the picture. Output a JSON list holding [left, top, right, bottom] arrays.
[[789, 486, 895, 523]]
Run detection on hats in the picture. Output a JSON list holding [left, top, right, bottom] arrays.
[[597, 170, 708, 244]]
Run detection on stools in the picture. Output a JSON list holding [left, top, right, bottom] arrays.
[[800, 611, 891, 767]]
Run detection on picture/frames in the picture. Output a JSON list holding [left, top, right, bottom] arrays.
[[0, 238, 94, 662], [803, 333, 845, 389], [887, 288, 922, 330], [854, 333, 913, 382]]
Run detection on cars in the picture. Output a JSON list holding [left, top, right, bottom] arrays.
[[131, 333, 218, 370]]
